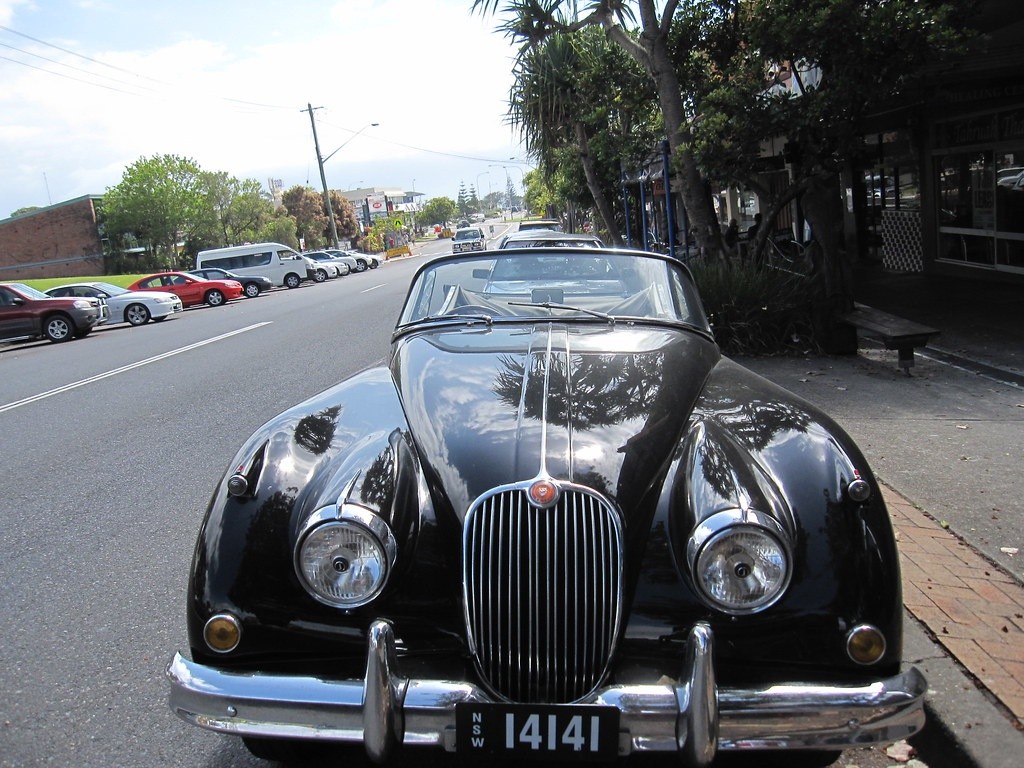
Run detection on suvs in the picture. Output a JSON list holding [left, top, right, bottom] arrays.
[[0, 283, 112, 343], [451, 227, 487, 253]]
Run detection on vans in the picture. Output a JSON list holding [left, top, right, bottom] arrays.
[[195, 243, 320, 289]]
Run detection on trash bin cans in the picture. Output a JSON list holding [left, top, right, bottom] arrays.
[[442, 228, 452, 238]]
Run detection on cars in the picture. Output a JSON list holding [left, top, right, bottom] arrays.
[[260, 251, 384, 283], [996, 166, 1024, 190], [473, 218, 624, 311], [165, 247, 929, 768], [125, 268, 274, 306], [41, 282, 183, 325], [456, 220, 471, 229]]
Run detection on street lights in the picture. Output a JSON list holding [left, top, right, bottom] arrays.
[[318, 123, 379, 249], [476, 171, 493, 213], [412, 179, 417, 190], [348, 180, 364, 191], [487, 157, 540, 210]]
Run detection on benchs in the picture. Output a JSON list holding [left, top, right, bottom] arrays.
[[839, 300, 941, 379]]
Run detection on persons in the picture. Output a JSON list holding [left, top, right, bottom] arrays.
[[388, 237, 394, 249], [747, 213, 762, 242], [725, 218, 739, 246], [404, 233, 415, 245]]
